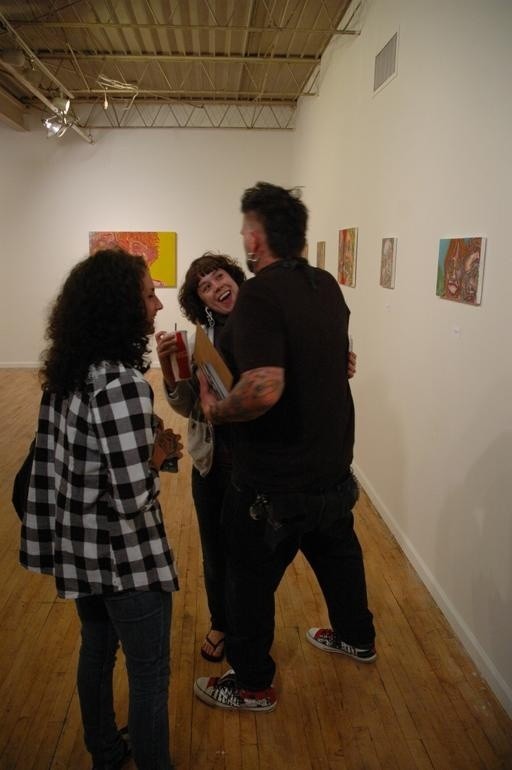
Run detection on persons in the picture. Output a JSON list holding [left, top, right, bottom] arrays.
[[153, 251, 358, 663], [194, 183, 376, 711], [17, 245, 184, 770]]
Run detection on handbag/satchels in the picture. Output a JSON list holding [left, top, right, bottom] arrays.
[[187, 397, 214, 479]]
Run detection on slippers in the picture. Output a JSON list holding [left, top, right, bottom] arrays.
[[201, 630, 225, 661]]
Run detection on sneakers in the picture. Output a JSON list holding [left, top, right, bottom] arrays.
[[193, 669, 279, 712], [306, 628, 377, 663]]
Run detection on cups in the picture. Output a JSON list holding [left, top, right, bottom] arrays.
[[166, 330, 193, 382]]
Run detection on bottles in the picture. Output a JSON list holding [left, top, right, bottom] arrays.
[[175, 332, 191, 379]]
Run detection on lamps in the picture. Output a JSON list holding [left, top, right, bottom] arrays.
[[41, 89, 71, 137]]
